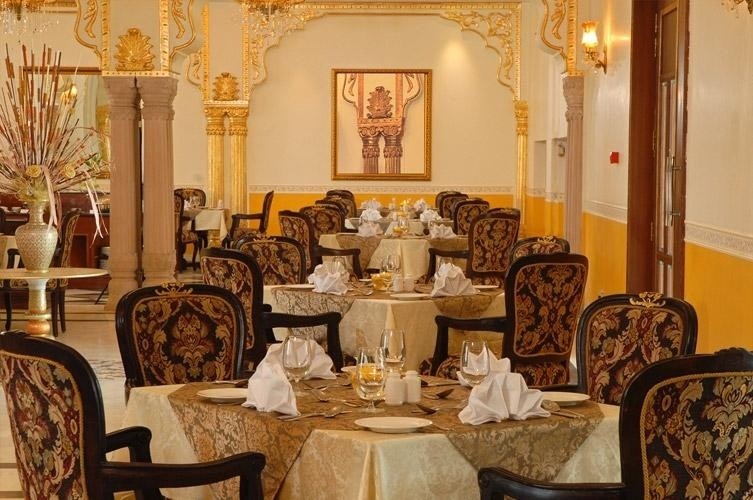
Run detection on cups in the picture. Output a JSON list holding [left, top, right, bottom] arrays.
[[218, 199, 223, 208]]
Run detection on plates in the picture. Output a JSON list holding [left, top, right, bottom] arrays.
[[543, 391, 591, 407], [200, 387, 249, 403], [286, 278, 502, 299], [355, 416, 433, 433], [341, 366, 356, 372], [338, 232, 356, 235]]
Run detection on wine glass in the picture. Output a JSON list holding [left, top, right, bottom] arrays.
[[379, 329, 405, 373], [461, 341, 489, 386], [357, 347, 385, 413], [193, 196, 199, 205], [283, 335, 311, 396], [360, 197, 439, 236], [330, 253, 452, 293]]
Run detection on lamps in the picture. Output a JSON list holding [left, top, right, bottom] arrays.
[[231, 1, 308, 36], [0, 1, 61, 56], [581, 21, 607, 76]]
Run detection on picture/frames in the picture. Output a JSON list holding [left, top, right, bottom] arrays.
[[329, 67, 432, 181]]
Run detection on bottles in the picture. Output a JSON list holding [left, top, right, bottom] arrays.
[[385, 370, 421, 406]]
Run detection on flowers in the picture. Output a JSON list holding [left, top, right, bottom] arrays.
[[0, 42, 110, 247]]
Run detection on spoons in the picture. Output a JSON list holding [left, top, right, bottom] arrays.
[[416, 388, 463, 414], [280, 389, 361, 423]]
